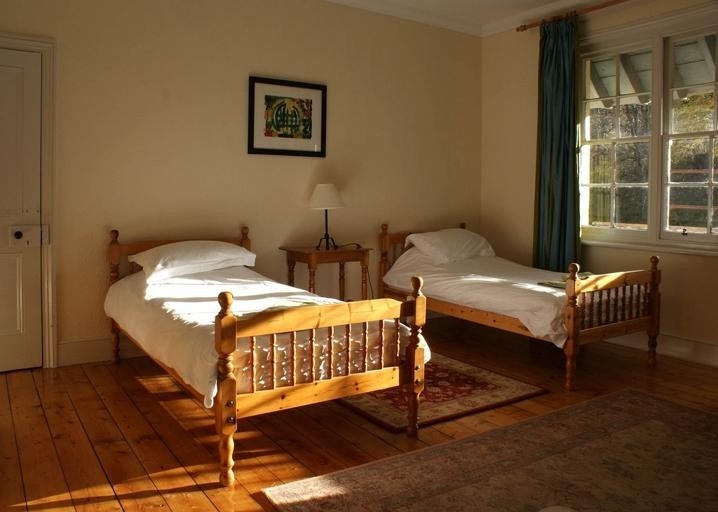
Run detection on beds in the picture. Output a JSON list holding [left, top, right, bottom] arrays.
[[109, 225, 428, 487], [378, 222, 662, 391]]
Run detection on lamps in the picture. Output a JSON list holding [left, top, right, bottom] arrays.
[[308, 183, 345, 250]]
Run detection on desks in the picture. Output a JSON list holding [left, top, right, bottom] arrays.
[[278, 245, 374, 302]]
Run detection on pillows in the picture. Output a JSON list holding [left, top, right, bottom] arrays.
[[404, 228, 496, 266], [127, 240, 257, 285]]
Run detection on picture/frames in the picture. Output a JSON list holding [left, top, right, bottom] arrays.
[[247, 75, 327, 159]]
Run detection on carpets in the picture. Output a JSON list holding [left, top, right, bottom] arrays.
[[259, 386, 717, 511], [331, 350, 552, 434]]
